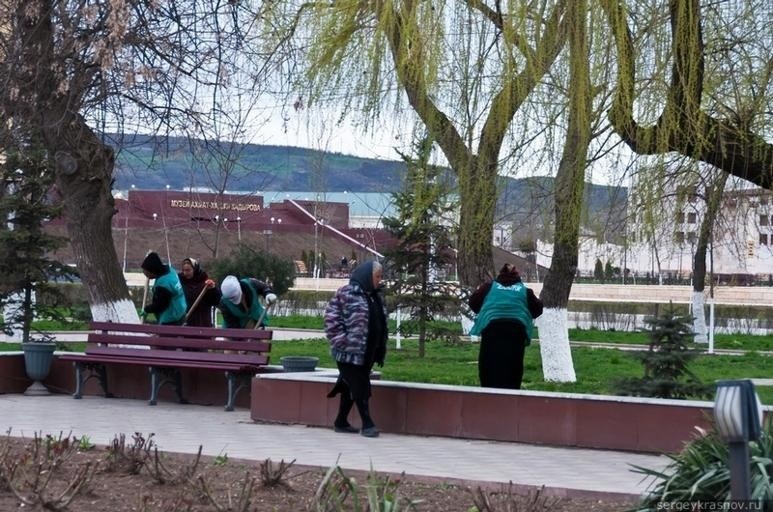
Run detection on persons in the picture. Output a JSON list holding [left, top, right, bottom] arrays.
[[178, 257, 221, 352], [139, 252, 187, 350], [468, 263, 543, 389], [220, 275, 277, 356], [324, 259, 388, 437]]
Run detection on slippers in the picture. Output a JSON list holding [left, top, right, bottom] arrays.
[[334, 423, 380, 437]]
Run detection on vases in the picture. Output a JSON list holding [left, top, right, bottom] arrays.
[[281, 355, 320, 373], [20, 341, 57, 396]]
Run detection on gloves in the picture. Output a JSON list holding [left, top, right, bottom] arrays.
[[205, 279, 216, 288], [265, 293, 277, 305]]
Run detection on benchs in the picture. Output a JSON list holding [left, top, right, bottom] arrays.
[[58, 319, 277, 412]]
